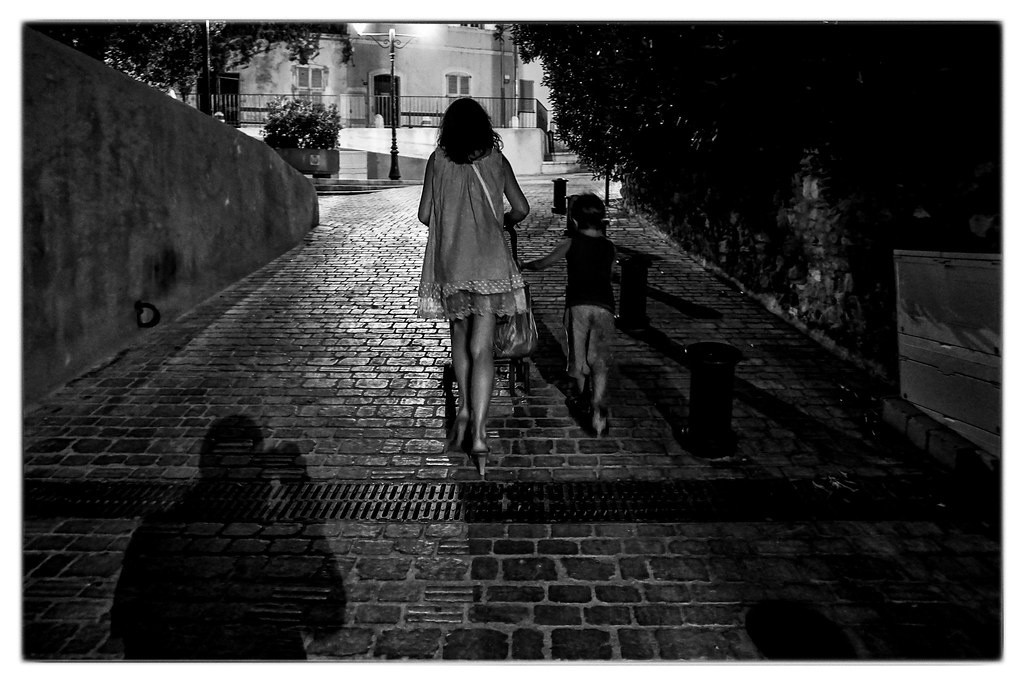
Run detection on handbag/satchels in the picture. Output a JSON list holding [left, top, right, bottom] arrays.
[[492, 279, 538, 359]]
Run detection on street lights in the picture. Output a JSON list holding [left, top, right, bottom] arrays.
[[355, 23, 434, 180]]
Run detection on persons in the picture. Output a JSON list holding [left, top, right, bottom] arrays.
[[520, 190, 620, 433], [414, 97, 530, 474]]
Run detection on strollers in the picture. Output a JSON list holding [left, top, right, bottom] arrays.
[[442, 227, 532, 395]]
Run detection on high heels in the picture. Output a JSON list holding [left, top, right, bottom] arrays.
[[470, 439, 489, 476], [449, 416, 470, 448]]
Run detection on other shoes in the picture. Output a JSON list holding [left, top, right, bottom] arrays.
[[576, 378, 587, 394], [592, 415, 607, 438]]
